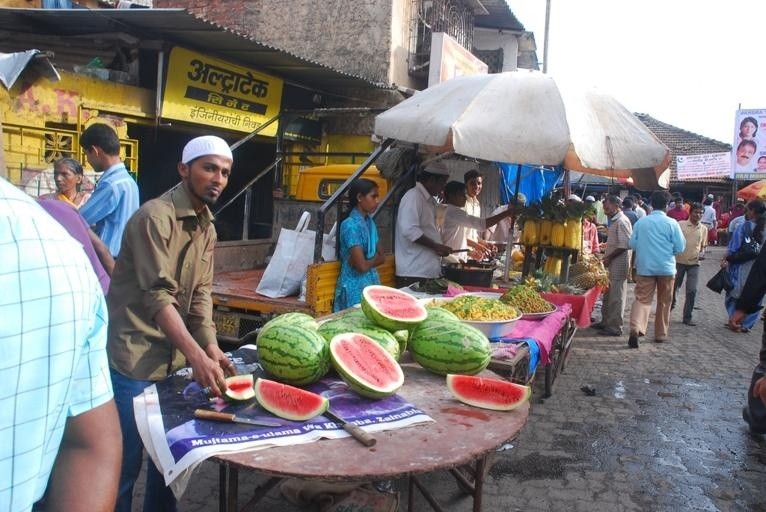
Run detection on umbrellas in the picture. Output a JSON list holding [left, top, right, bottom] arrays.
[[372, 70, 673, 283]]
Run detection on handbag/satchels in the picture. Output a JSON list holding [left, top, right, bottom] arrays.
[[255, 228, 318, 298], [299, 234, 340, 302], [726, 233, 760, 264]]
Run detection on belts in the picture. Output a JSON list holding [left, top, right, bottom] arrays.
[[702, 222, 712, 225]]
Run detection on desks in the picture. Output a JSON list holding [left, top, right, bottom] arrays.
[[153, 349, 531, 512]]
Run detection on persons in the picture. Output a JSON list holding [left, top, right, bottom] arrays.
[[105, 136, 238, 512], [331, 176, 387, 312], [75, 123, 140, 260], [735, 139, 757, 167], [570, 191, 766, 348], [0, 176, 124, 511], [393, 162, 526, 284], [25, 193, 115, 302], [738, 116, 759, 143], [38, 156, 97, 211], [741, 307, 766, 435]]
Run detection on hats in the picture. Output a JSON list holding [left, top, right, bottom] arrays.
[[181, 136, 234, 165], [586, 196, 595, 201], [565, 194, 582, 203], [424, 160, 449, 176]]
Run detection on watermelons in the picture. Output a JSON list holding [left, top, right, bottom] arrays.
[[252, 379, 331, 422], [343, 310, 409, 354], [220, 374, 255, 403], [256, 312, 318, 349], [361, 285, 427, 330], [405, 321, 491, 376], [330, 333, 405, 399], [315, 317, 401, 368], [447, 374, 532, 410], [419, 306, 459, 322], [259, 326, 329, 386]]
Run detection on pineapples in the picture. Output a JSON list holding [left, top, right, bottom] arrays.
[[516, 198, 605, 293]]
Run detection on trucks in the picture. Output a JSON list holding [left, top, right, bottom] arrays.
[[181, 164, 396, 344]]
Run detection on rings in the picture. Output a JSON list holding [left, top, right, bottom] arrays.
[[226, 362, 234, 369]]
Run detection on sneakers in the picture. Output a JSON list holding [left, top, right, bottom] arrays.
[[628, 328, 640, 348], [683, 319, 696, 326], [590, 321, 606, 330], [655, 334, 667, 342], [598, 327, 622, 336]]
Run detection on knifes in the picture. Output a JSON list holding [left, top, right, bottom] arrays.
[[452, 248, 469, 252], [323, 409, 376, 446], [195, 409, 281, 427]]
